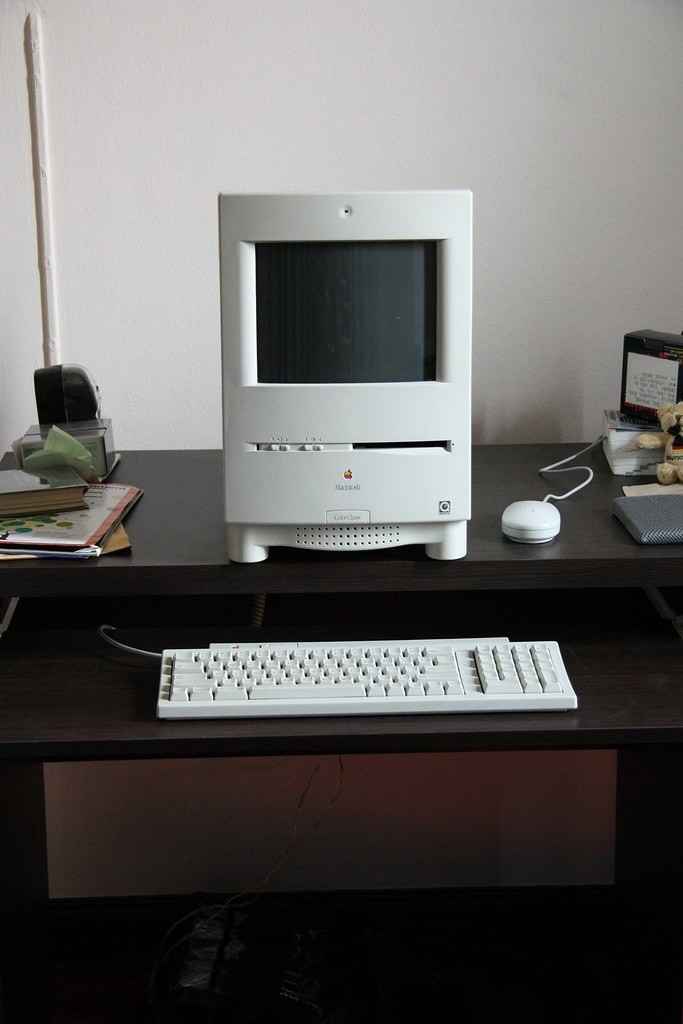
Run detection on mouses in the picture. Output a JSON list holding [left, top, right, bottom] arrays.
[[503, 501, 561, 543]]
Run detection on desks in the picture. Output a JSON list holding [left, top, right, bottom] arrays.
[[0, 439, 683, 1024]]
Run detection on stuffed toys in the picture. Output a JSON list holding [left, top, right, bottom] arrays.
[[634, 400, 683, 485]]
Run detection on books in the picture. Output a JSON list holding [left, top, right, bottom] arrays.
[[0, 465, 145, 558]]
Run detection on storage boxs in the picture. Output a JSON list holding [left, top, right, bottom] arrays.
[[620, 328, 683, 425], [10, 418, 116, 478]]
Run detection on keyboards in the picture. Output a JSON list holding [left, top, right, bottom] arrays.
[[157, 636, 578, 719]]
